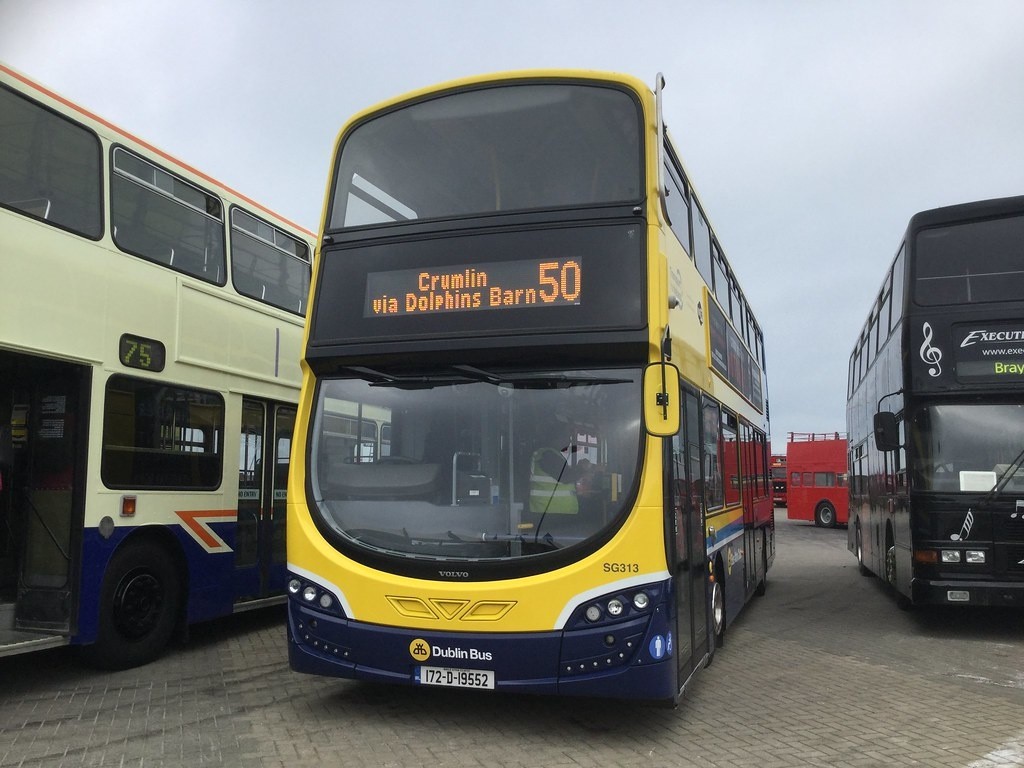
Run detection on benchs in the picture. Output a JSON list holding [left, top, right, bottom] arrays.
[[6, 199, 302, 314], [254, 458, 289, 483]]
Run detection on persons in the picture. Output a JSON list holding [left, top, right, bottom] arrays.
[[529, 424, 593, 514]]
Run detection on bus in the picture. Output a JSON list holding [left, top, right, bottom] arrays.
[[769, 461, 786, 508], [280, 67, 776, 713], [0, 64, 395, 672], [842, 194, 1024, 614], [786, 432, 850, 527]]
